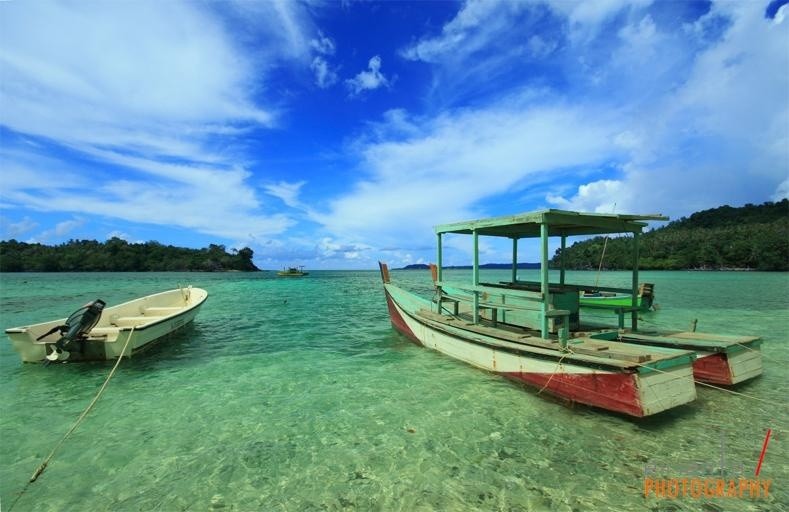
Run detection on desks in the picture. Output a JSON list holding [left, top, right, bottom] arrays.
[[474, 280, 580, 334]]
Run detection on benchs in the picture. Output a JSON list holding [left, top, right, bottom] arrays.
[[141, 306, 180, 315], [440, 293, 571, 342], [116, 316, 157, 325], [579, 302, 646, 330]]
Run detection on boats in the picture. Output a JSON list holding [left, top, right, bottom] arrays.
[[579, 203, 656, 312], [377, 207, 766, 419], [5, 284, 210, 364], [276, 265, 310, 277]]
